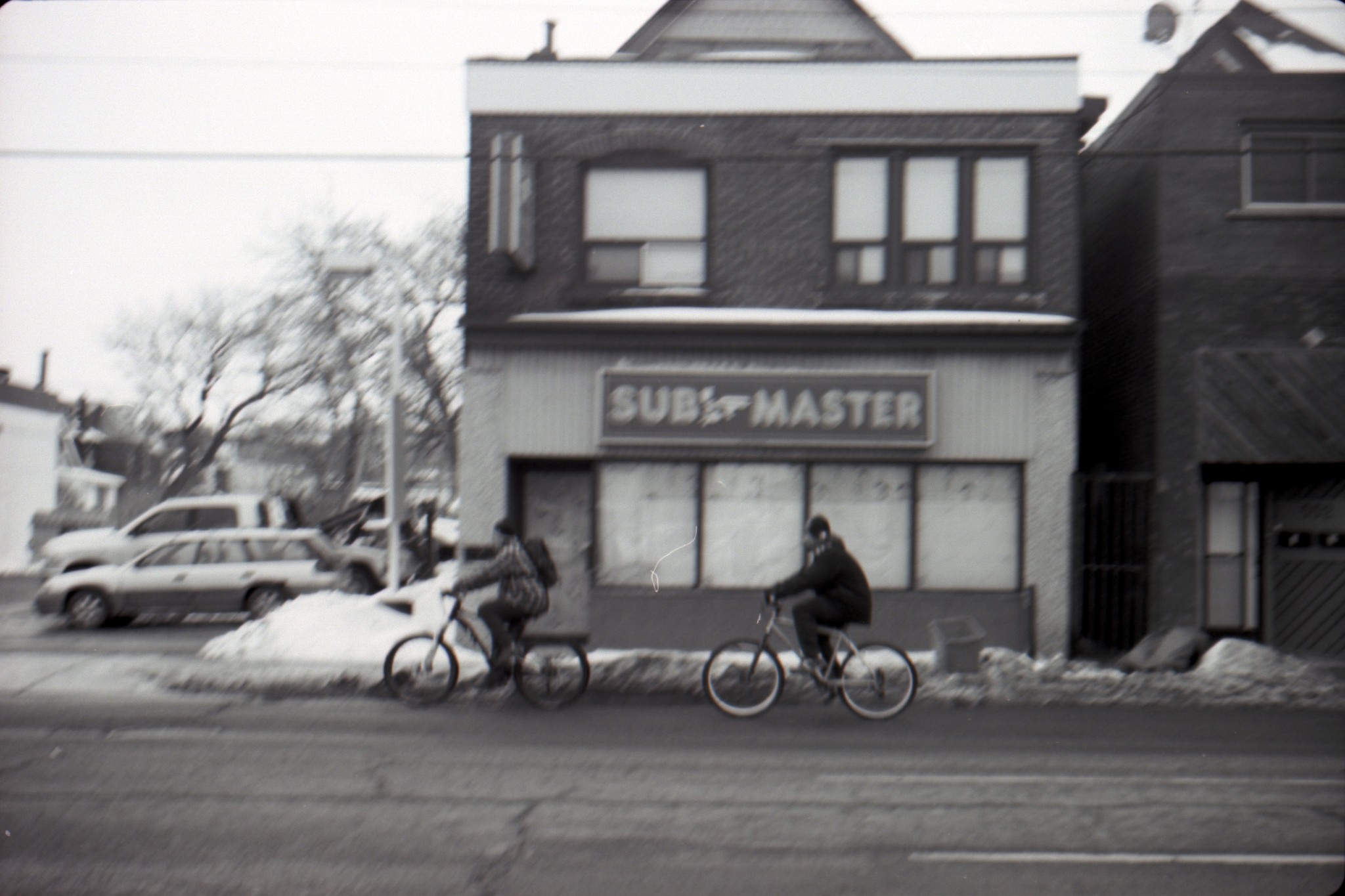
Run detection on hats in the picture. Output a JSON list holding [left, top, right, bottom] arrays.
[[495, 518, 516, 535], [807, 516, 829, 539]]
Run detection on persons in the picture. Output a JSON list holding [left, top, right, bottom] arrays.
[[452, 517, 549, 688], [759, 514, 873, 700], [398, 519, 437, 589]]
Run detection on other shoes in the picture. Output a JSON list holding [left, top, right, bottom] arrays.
[[790, 657, 825, 674], [820, 690, 836, 706]]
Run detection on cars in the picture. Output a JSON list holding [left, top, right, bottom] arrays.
[[36, 526, 356, 632], [334, 482, 461, 565]]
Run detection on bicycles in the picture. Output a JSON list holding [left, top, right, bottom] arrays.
[[702, 594, 919, 721], [383, 589, 592, 711]]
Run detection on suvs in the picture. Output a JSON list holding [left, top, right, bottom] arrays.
[[37, 494, 288, 580]]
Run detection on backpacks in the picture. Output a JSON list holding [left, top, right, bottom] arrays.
[[524, 538, 556, 587]]
